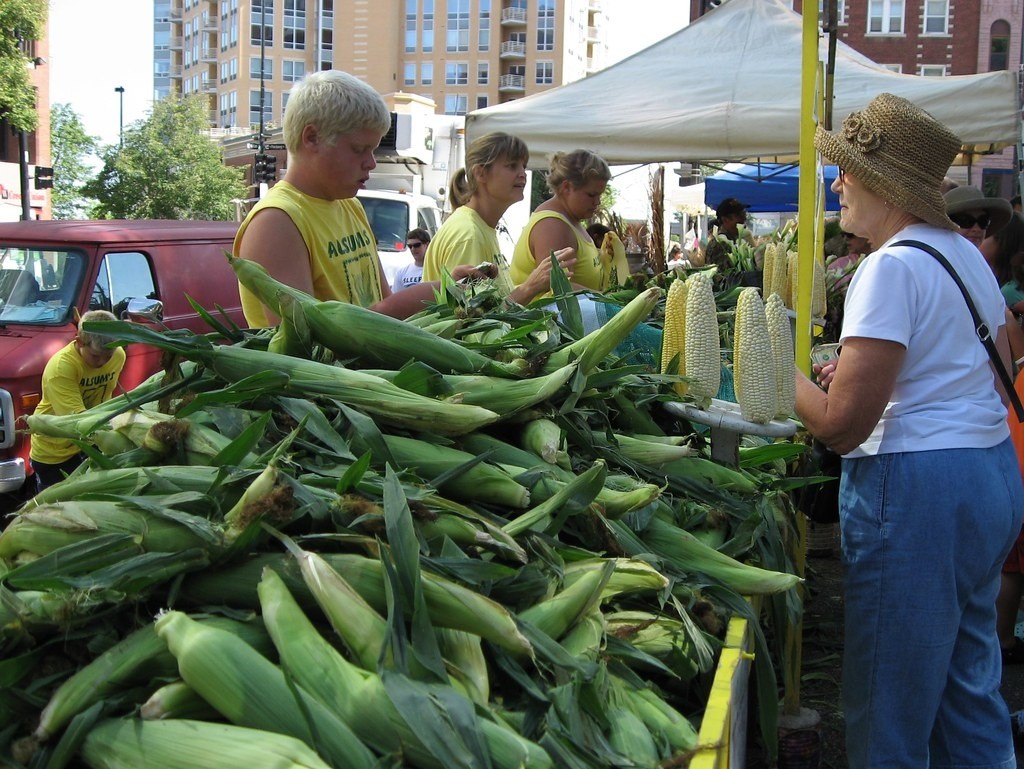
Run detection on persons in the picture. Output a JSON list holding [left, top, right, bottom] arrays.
[[942, 185, 1013, 251], [231, 71, 497, 330], [586, 224, 611, 249], [708, 219, 719, 241], [827, 228, 876, 289], [792, 91, 1024, 769], [980, 196, 1023, 478], [28, 311, 126, 492], [705, 236, 739, 292], [716, 198, 751, 245], [510, 149, 615, 301], [420, 133, 578, 314], [391, 228, 431, 294], [668, 244, 691, 269]]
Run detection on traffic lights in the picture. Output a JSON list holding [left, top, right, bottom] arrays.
[[265, 154, 277, 182], [254, 153, 265, 183]]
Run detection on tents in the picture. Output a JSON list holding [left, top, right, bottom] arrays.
[[465, 0, 1024, 170], [703, 163, 840, 249]]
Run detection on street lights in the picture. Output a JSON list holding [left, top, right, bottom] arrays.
[[114, 87, 126, 152]]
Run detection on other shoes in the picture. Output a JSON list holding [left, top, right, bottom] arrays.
[[1000, 636, 1024, 667]]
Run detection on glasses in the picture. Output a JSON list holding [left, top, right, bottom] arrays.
[[835, 164, 846, 181], [408, 242, 425, 249], [950, 210, 991, 230]]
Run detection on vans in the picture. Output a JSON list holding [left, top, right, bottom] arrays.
[[0, 218, 246, 507], [352, 187, 444, 298]]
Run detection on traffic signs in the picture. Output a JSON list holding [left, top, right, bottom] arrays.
[[265, 143, 286, 150]]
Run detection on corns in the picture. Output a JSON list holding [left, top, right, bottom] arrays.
[[0, 239, 828, 769]]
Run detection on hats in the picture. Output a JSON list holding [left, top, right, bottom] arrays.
[[718, 196, 752, 218], [943, 185, 1012, 239], [812, 93, 961, 231]]
